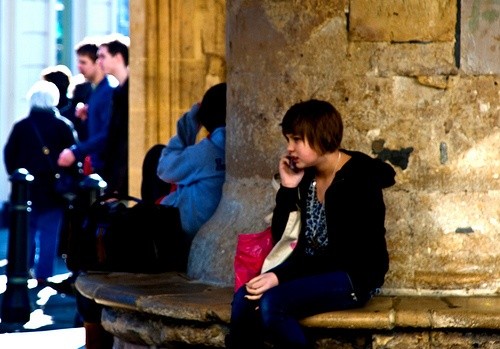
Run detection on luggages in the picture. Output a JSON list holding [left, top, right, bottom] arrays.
[[8, 179, 36, 270]]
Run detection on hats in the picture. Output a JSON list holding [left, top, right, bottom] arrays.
[[32, 80, 60, 108], [199, 83, 226, 134]]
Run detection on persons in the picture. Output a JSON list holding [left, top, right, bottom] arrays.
[[4, 81, 79, 285], [225, 99, 397, 349], [96, 39, 129, 199], [39, 39, 113, 287], [76, 82, 227, 349]]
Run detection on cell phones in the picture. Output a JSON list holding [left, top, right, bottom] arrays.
[[289, 158, 299, 173]]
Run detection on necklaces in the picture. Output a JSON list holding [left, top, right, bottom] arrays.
[[310, 151, 341, 239]]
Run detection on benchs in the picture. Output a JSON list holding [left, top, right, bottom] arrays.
[[75, 269, 500, 349]]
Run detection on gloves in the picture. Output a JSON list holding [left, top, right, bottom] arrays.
[[177, 102, 204, 147]]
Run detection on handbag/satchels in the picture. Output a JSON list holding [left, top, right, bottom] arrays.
[[74, 194, 146, 275], [260, 173, 301, 274], [234, 227, 273, 293], [53, 172, 73, 194]]
